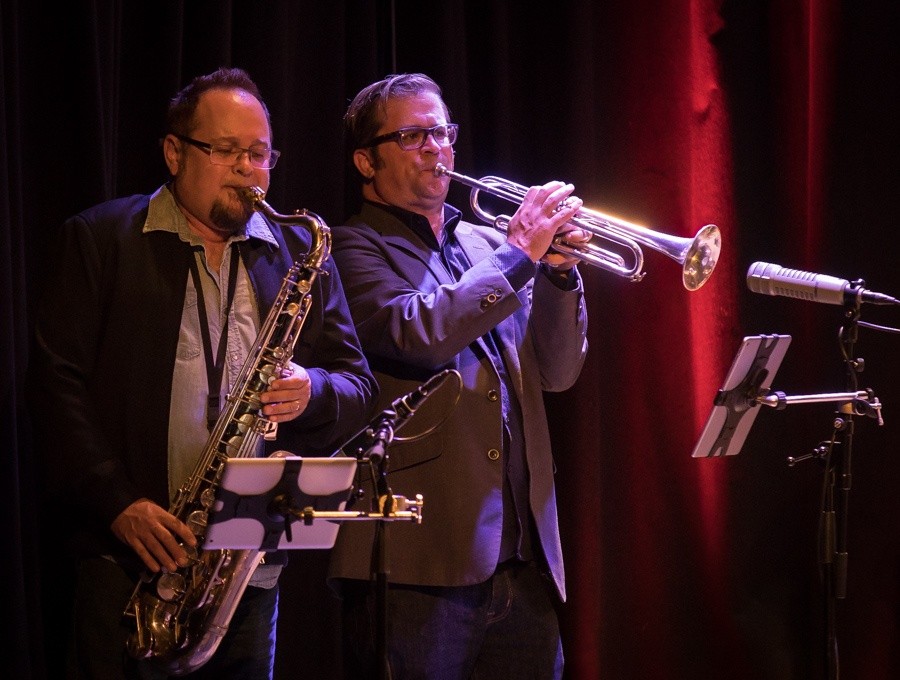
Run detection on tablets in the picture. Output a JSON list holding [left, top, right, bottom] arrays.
[[692, 336, 791, 458], [201, 456, 358, 551]]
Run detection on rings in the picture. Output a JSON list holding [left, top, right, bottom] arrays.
[[296, 401, 299, 410]]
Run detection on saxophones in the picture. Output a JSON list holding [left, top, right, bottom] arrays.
[[125, 188, 333, 676]]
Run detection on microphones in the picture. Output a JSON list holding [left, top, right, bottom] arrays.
[[746, 261, 900, 306], [330, 369, 450, 459]]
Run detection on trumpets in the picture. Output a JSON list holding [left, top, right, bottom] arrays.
[[433, 162, 722, 292]]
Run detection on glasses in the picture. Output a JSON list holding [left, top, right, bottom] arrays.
[[174, 133, 280, 171], [363, 122, 459, 152]]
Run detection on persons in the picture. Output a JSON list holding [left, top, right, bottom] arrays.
[[326, 72, 589, 679], [36, 70, 380, 679]]
